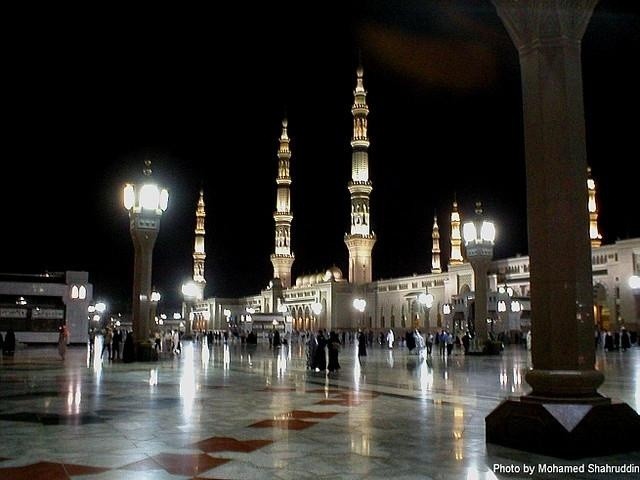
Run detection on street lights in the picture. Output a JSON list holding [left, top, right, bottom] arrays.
[[124, 160, 169, 343], [180, 281, 197, 339], [459, 201, 495, 354], [418, 286, 433, 330]]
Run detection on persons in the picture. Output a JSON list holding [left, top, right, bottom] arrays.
[[268, 329, 281, 350], [357, 332, 367, 365], [88, 328, 180, 360], [520, 325, 532, 351], [57, 324, 69, 361], [306, 329, 341, 376], [386, 325, 473, 356], [594, 321, 631, 352]]
